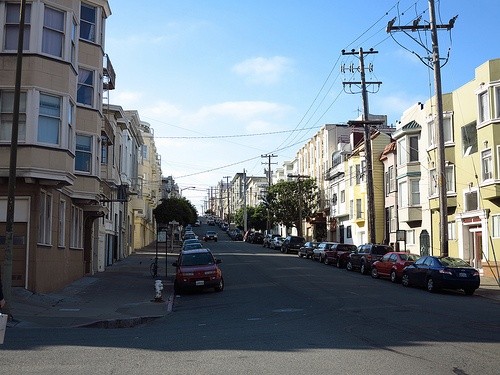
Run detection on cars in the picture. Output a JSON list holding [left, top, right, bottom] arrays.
[[310, 241, 342, 263], [203, 230, 219, 242], [269, 236, 286, 250], [207, 220, 216, 226], [297, 241, 323, 260], [193, 219, 200, 227], [323, 243, 358, 269], [182, 225, 196, 239], [213, 215, 243, 240], [183, 238, 200, 246], [401, 255, 481, 297], [248, 232, 264, 244], [371, 251, 422, 284], [181, 243, 204, 251], [171, 247, 225, 294]]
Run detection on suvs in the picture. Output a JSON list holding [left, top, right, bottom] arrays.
[[262, 233, 282, 249], [279, 235, 306, 254], [343, 244, 395, 276]]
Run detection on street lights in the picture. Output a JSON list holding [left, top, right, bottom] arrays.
[[180, 186, 196, 198]]
